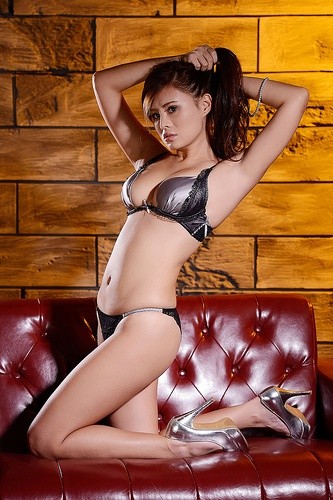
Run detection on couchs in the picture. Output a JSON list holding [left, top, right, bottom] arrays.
[[0, 292, 333, 500]]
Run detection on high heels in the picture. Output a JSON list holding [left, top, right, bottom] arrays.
[[163, 397, 248, 452], [258, 386, 312, 439]]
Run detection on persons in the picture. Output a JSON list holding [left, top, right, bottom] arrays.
[[26, 43, 313, 459]]
